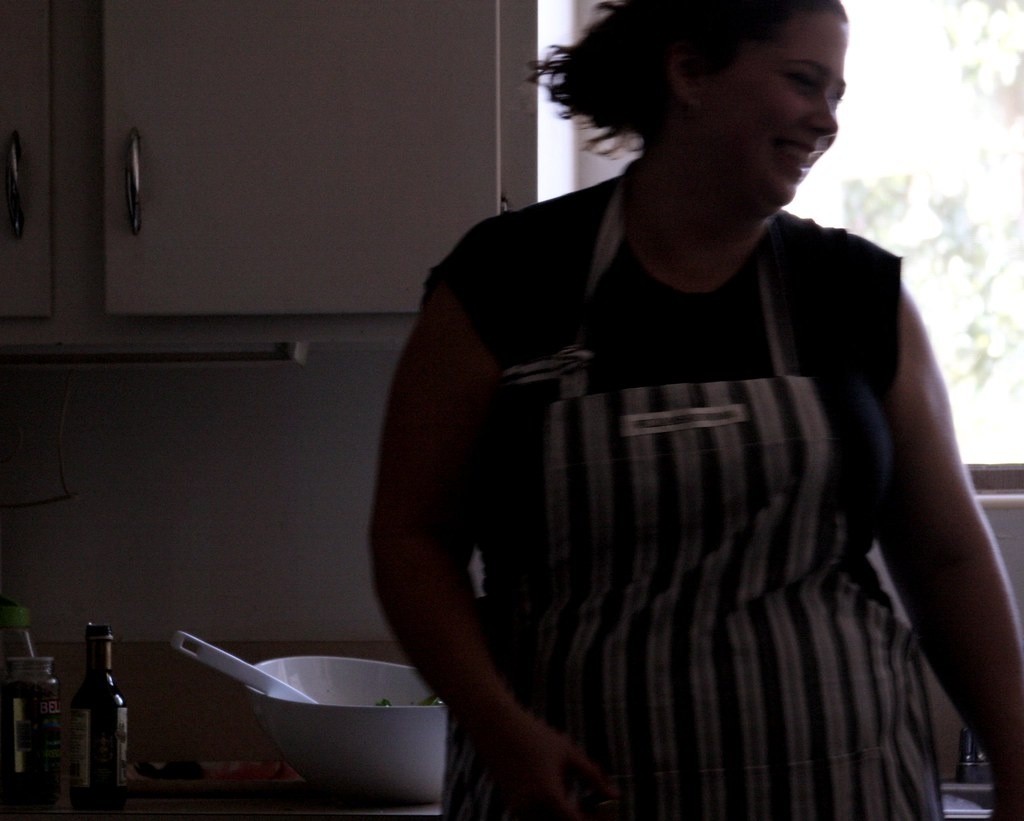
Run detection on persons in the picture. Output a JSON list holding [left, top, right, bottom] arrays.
[[369, 0, 1024, 821]]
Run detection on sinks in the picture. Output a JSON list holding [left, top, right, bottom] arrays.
[[944, 787, 998, 811]]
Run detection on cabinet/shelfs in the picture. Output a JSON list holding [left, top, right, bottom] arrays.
[[0, 0, 539, 366]]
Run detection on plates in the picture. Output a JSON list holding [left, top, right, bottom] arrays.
[[127, 761, 305, 792]]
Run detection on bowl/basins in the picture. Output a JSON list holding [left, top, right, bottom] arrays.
[[245, 656, 449, 804]]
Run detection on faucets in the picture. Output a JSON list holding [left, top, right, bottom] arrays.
[[956, 724, 997, 783]]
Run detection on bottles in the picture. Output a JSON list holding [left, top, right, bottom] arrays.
[[0, 594, 32, 679], [70, 623, 128, 811], [0, 655, 62, 808]]
